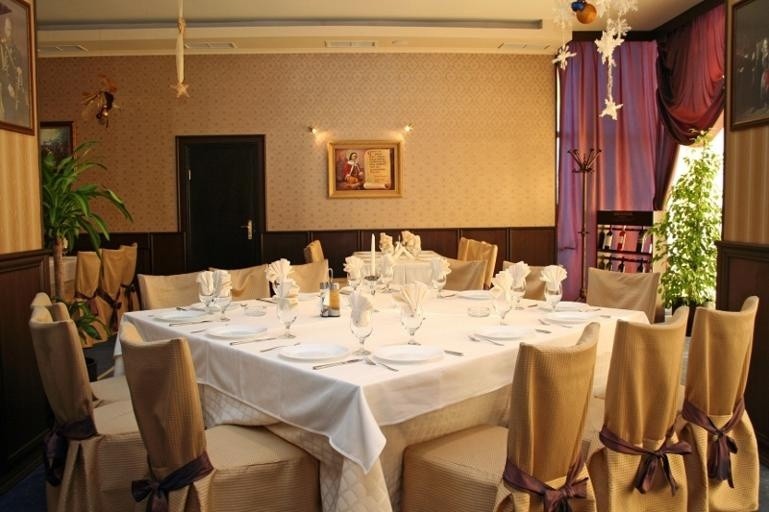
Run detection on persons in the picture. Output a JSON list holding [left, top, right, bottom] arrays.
[[0, 16, 30, 126], [342, 150, 362, 184]]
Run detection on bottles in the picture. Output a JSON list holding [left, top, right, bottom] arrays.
[[598, 223, 652, 273]]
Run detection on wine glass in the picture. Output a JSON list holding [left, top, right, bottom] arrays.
[[198, 271, 564, 363]]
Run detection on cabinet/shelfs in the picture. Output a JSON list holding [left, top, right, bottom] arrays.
[[594, 209, 669, 322]]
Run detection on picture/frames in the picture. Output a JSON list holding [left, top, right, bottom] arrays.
[[39, 119, 77, 182], [326, 138, 404, 199], [729, 0, 769, 131], [0, 1, 36, 137]]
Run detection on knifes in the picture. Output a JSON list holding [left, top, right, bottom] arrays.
[[313, 358, 363, 370], [168, 320, 211, 326]]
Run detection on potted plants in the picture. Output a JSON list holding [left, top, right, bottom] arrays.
[[649, 127, 728, 336], [36, 141, 136, 383]]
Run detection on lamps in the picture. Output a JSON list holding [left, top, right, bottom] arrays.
[[551, 0, 639, 121]]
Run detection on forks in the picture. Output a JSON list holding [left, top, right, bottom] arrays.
[[365, 359, 399, 372]]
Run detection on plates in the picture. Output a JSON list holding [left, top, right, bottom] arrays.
[[542, 312, 591, 322], [149, 309, 204, 321], [558, 300, 586, 312]]
